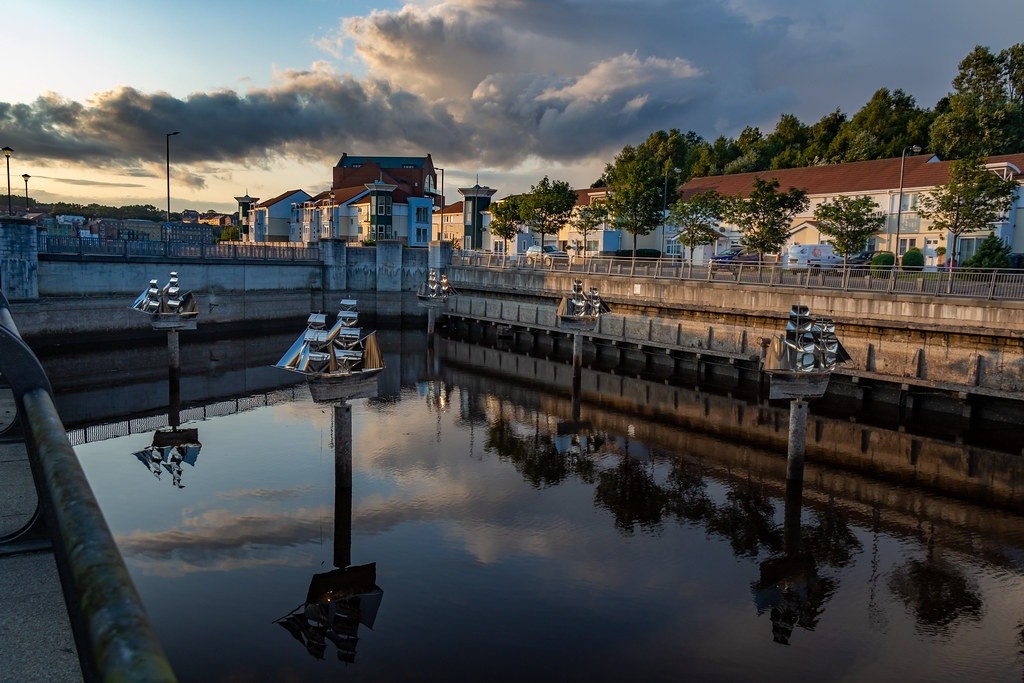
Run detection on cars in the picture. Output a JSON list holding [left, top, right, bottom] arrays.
[[833, 250, 900, 277]]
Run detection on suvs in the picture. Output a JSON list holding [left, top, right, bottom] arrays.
[[525, 245, 569, 268], [709, 248, 766, 270], [788, 244, 846, 269]]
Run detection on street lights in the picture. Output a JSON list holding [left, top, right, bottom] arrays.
[[892, 145, 924, 269], [660, 167, 681, 264], [433, 167, 445, 242], [2, 147, 20, 216], [21, 174, 32, 214], [328, 191, 337, 238], [166, 131, 181, 220]]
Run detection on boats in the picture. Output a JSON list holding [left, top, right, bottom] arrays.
[[131, 269, 201, 322], [758, 303, 855, 377], [272, 295, 390, 380], [416, 269, 460, 305], [762, 375, 837, 404], [148, 318, 200, 331], [557, 277, 612, 323], [131, 425, 202, 491], [305, 377, 381, 403]]
